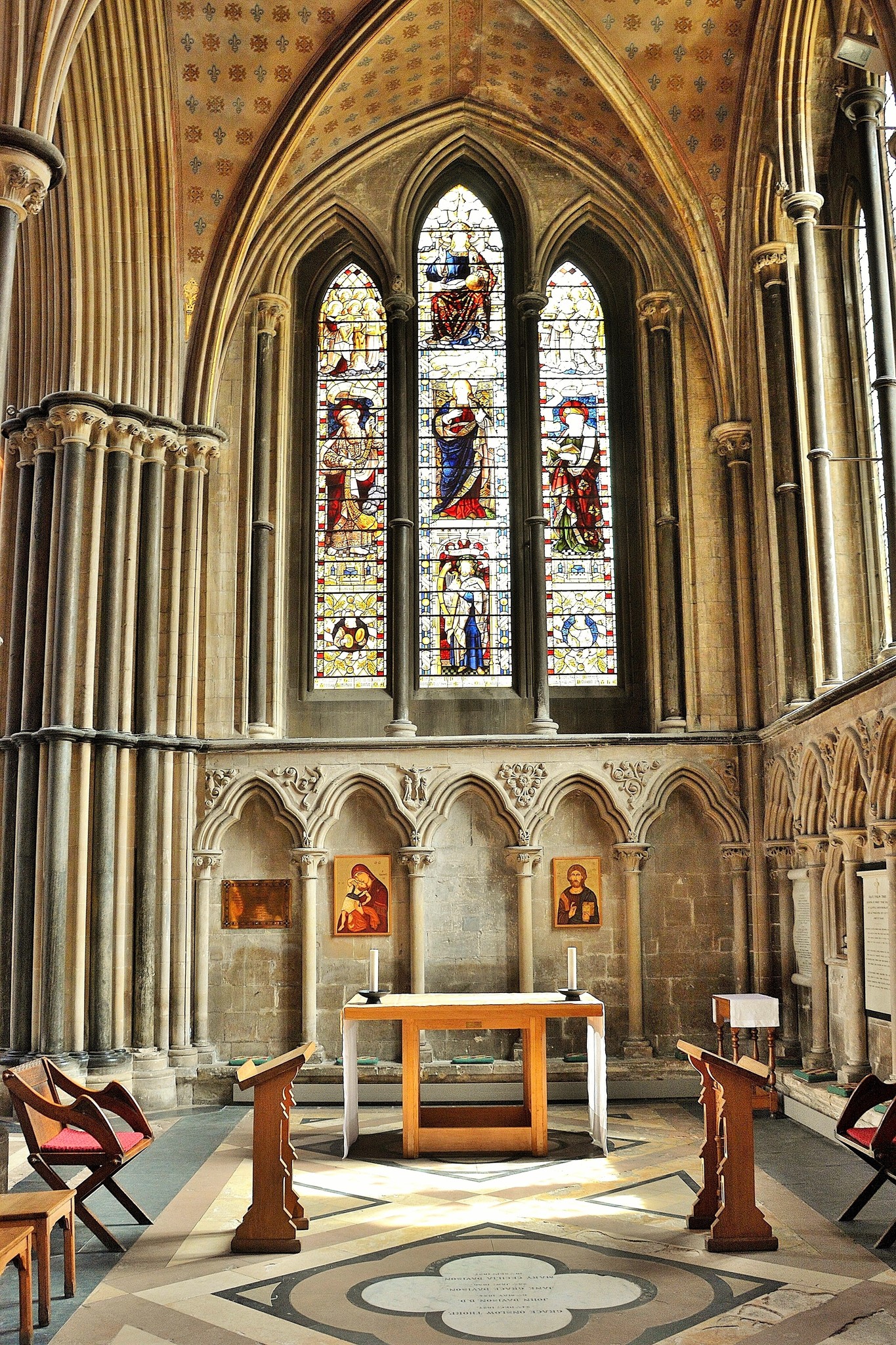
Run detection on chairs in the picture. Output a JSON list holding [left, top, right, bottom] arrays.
[[0, 1189, 78, 1324], [833, 1074, 895, 1248], [0, 1225, 34, 1345], [3, 1056, 155, 1253]]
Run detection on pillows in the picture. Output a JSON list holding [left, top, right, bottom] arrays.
[[792, 1066, 837, 1083], [675, 1051, 688, 1061], [451, 1054, 494, 1064], [775, 1055, 800, 1067], [875, 1098, 892, 1114], [564, 1052, 588, 1062], [827, 1082, 859, 1097], [229, 1055, 271, 1065], [336, 1056, 378, 1065]]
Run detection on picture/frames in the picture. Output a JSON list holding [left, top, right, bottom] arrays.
[[334, 855, 391, 935], [552, 856, 601, 927]]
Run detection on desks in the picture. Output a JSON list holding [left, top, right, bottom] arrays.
[[344, 990, 602, 1159]]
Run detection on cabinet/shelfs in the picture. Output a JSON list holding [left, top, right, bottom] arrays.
[[712, 994, 780, 1119]]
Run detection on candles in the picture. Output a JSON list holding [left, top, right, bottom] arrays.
[[369, 949, 378, 992], [567, 947, 578, 990]]
